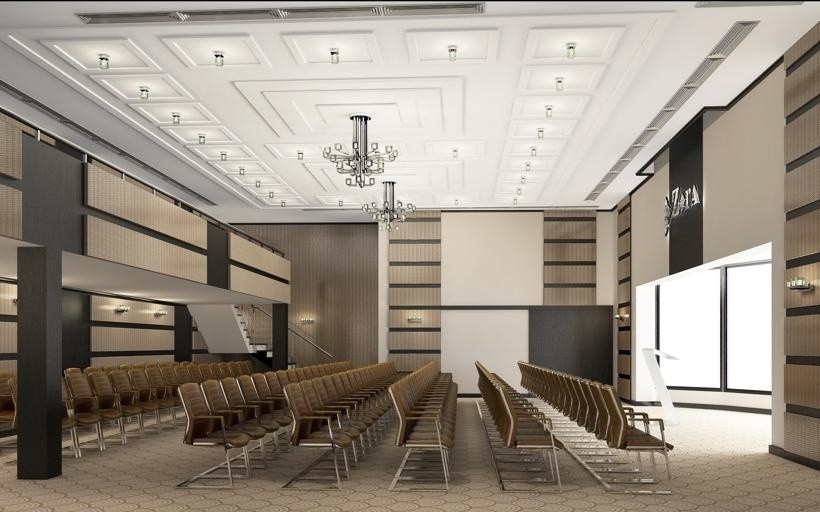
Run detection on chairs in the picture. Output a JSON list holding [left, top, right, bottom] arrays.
[[0, 373, 17, 433], [61, 374, 78, 460], [280, 362, 412, 489], [67, 372, 101, 459], [63, 368, 82, 376], [519, 363, 675, 497], [387, 361, 459, 495], [475, 361, 581, 493], [177, 358, 355, 490], [84, 358, 254, 497]]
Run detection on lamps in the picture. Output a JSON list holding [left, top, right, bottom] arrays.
[[153, 309, 168, 318], [518, 188, 522, 196], [256, 181, 261, 187], [215, 50, 223, 70], [452, 147, 459, 158], [269, 192, 273, 198], [97, 55, 110, 69], [221, 152, 227, 162], [199, 134, 205, 144], [454, 199, 459, 207], [787, 276, 815, 296], [139, 87, 148, 100], [328, 50, 340, 65], [556, 77, 564, 92], [448, 44, 461, 63], [521, 176, 527, 185], [113, 304, 130, 315], [565, 43, 576, 61], [513, 198, 517, 206], [526, 162, 532, 172], [537, 128, 544, 139], [323, 115, 399, 187], [406, 316, 426, 324], [172, 112, 179, 124], [298, 153, 303, 160], [614, 314, 629, 322], [531, 146, 538, 156], [546, 105, 553, 118], [339, 201, 342, 206], [299, 317, 315, 325], [281, 202, 285, 207], [239, 168, 245, 175], [360, 181, 418, 237]]
